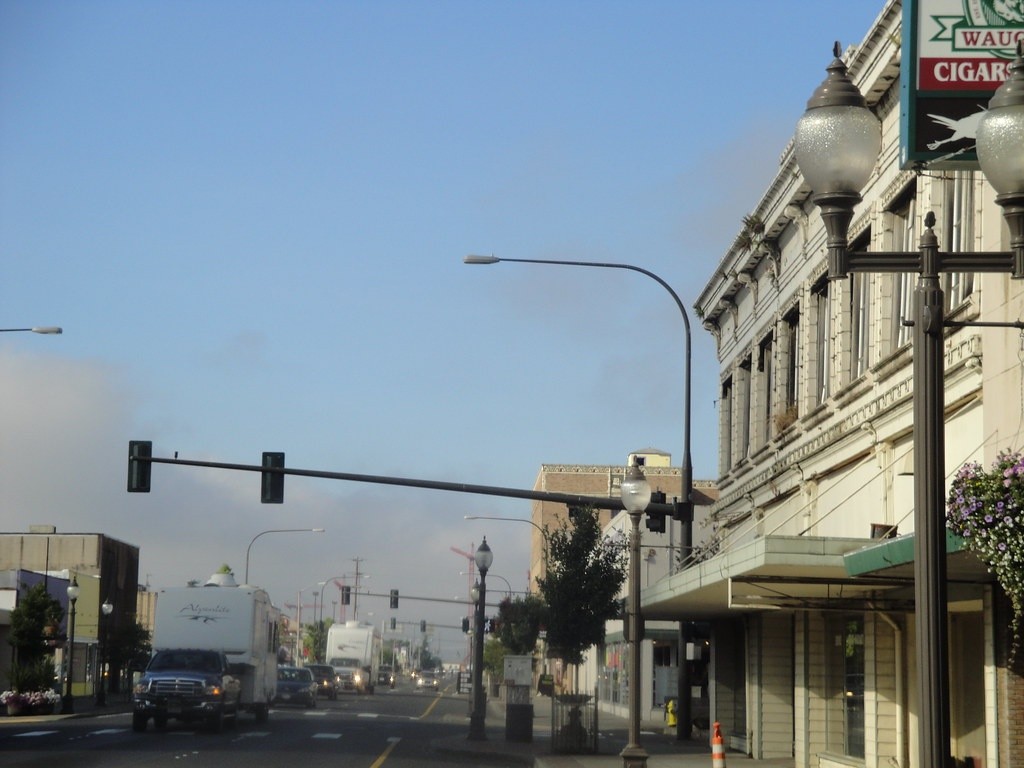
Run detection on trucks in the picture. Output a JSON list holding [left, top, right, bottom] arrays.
[[326, 619, 382, 696], [131, 576, 283, 734]]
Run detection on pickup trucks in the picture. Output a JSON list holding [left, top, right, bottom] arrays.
[[307, 665, 341, 700]]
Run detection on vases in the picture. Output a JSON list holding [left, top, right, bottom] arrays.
[[5, 656, 59, 715]]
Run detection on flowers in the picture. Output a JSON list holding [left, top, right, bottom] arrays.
[[0, 686, 63, 706], [944, 445, 1024, 671]]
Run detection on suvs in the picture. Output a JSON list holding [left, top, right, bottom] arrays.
[[378, 665, 396, 688]]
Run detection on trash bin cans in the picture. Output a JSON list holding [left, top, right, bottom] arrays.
[[503, 704, 533, 744]]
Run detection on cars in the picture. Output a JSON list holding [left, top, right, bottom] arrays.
[[272, 666, 318, 709], [417, 671, 439, 691]]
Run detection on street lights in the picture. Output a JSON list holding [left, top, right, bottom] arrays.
[[94, 594, 115, 707], [61, 576, 80, 713], [460, 513, 551, 677], [318, 572, 367, 664], [795, 34, 1024, 768], [621, 451, 652, 768], [462, 536, 495, 743], [245, 526, 326, 587], [464, 248, 698, 744]]
